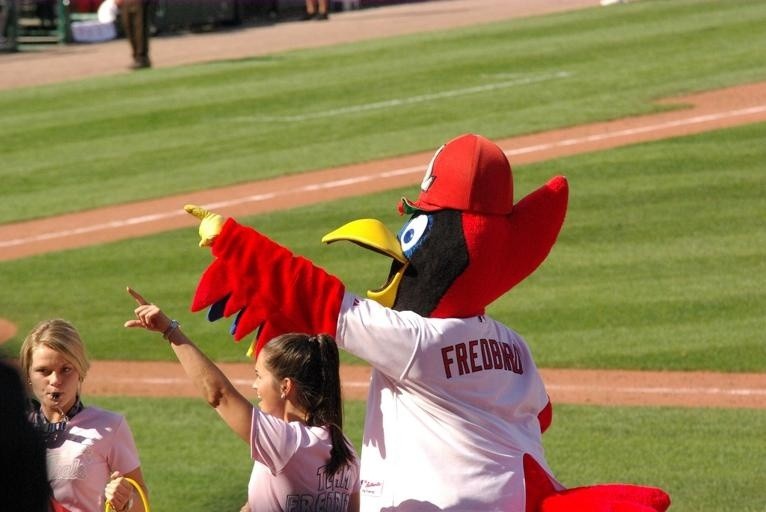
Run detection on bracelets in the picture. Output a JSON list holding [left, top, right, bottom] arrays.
[[162, 319, 179, 340], [118, 499, 131, 512]]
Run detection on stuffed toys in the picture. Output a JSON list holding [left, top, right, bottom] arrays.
[[182, 129, 671, 512]]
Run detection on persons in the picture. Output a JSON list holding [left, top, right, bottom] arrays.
[[297, 0, 329, 21], [17, 319, 150, 512], [96, 0, 152, 69], [0, 356, 51, 512], [122, 284, 361, 512]]
